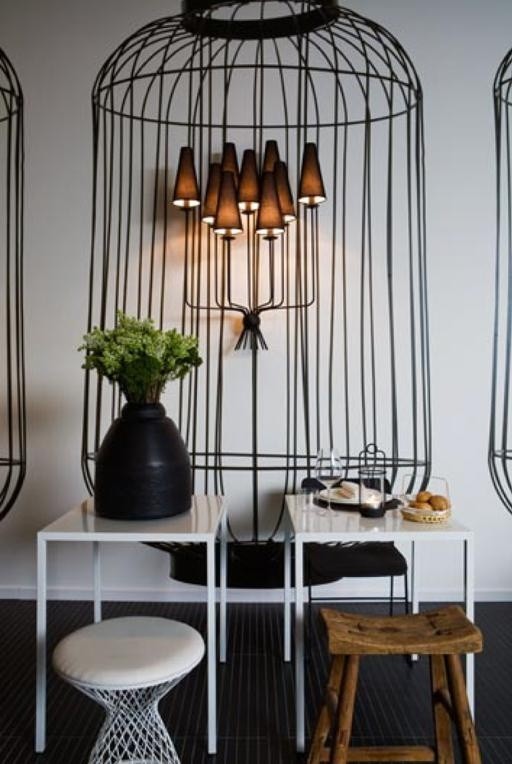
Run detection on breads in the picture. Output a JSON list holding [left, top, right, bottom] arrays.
[[410, 491, 448, 511]]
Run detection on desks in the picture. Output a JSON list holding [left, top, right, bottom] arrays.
[[35, 495, 230, 756], [282, 495, 477, 753]]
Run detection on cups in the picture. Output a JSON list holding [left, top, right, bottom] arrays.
[[292, 487, 322, 515]]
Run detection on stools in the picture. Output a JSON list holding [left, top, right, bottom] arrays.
[[306, 603, 485, 764], [52, 615, 207, 764]]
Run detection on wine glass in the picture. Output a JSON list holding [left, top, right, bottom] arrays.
[[312, 446, 348, 519]]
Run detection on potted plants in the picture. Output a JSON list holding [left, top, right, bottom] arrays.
[[76, 310, 193, 521]]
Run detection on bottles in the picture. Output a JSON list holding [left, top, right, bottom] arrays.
[[358, 446, 385, 519]]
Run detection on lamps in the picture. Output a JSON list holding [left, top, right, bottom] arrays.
[[78, 1, 433, 590]]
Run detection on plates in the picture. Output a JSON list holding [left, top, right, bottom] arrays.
[[319, 488, 391, 507], [398, 503, 454, 523]]
